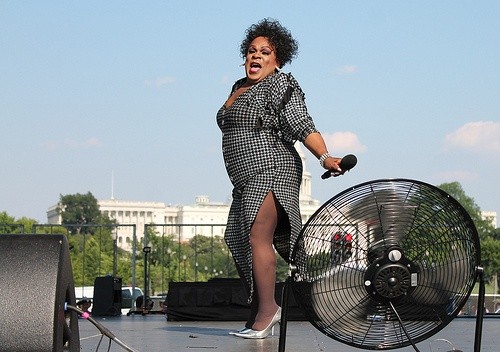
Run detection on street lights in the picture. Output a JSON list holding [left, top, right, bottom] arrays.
[[166, 247, 172, 283], [183, 255, 187, 282], [143, 245, 151, 314]]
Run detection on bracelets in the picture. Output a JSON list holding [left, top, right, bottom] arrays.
[[319, 152, 330, 168]]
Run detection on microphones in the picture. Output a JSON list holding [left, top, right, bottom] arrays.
[[321, 154, 357, 179]]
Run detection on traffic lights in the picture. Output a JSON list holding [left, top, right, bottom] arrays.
[[331, 232, 342, 265], [342, 233, 353, 263]]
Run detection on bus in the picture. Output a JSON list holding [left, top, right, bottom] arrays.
[[451, 293, 500, 318]]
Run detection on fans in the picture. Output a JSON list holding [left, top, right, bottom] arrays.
[[278, 179, 486, 352]]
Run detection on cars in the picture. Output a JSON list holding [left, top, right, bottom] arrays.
[[74, 284, 167, 315]]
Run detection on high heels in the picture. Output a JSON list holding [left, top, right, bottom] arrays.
[[235, 305, 284, 338], [228, 324, 275, 336]]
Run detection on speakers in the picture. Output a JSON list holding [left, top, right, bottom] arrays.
[[0, 233, 80, 352], [92, 276, 122, 316]]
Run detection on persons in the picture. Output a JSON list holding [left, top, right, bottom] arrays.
[[216, 18, 350, 339]]
